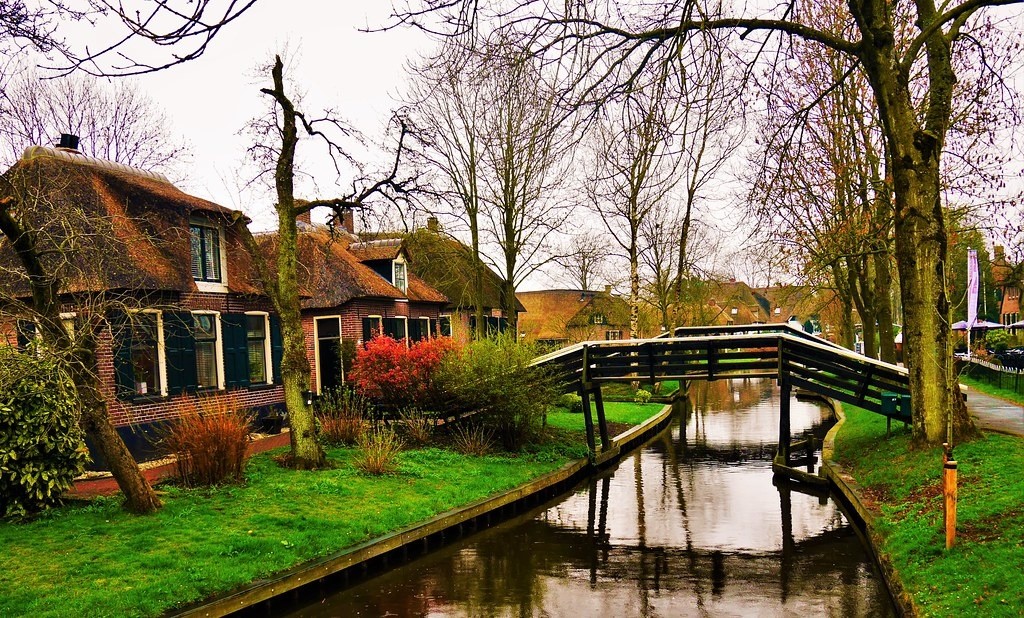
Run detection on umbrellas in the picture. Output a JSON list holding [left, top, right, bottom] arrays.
[[952, 319, 1005, 344], [1005, 320, 1024, 329]]
